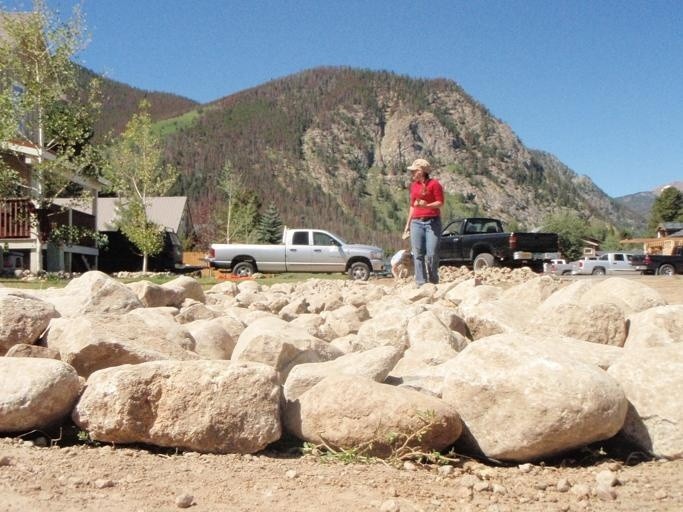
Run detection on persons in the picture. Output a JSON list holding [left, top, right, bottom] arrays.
[[401, 158, 444, 290], [389, 248, 414, 283]]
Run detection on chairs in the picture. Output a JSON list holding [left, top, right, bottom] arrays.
[[486, 225, 498, 231], [467, 225, 478, 233]]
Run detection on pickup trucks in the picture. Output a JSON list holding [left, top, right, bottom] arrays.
[[198, 225, 386, 281], [631, 245, 682, 277], [436, 217, 645, 277]]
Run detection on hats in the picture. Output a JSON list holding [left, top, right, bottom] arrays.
[[407, 159, 431, 174]]
